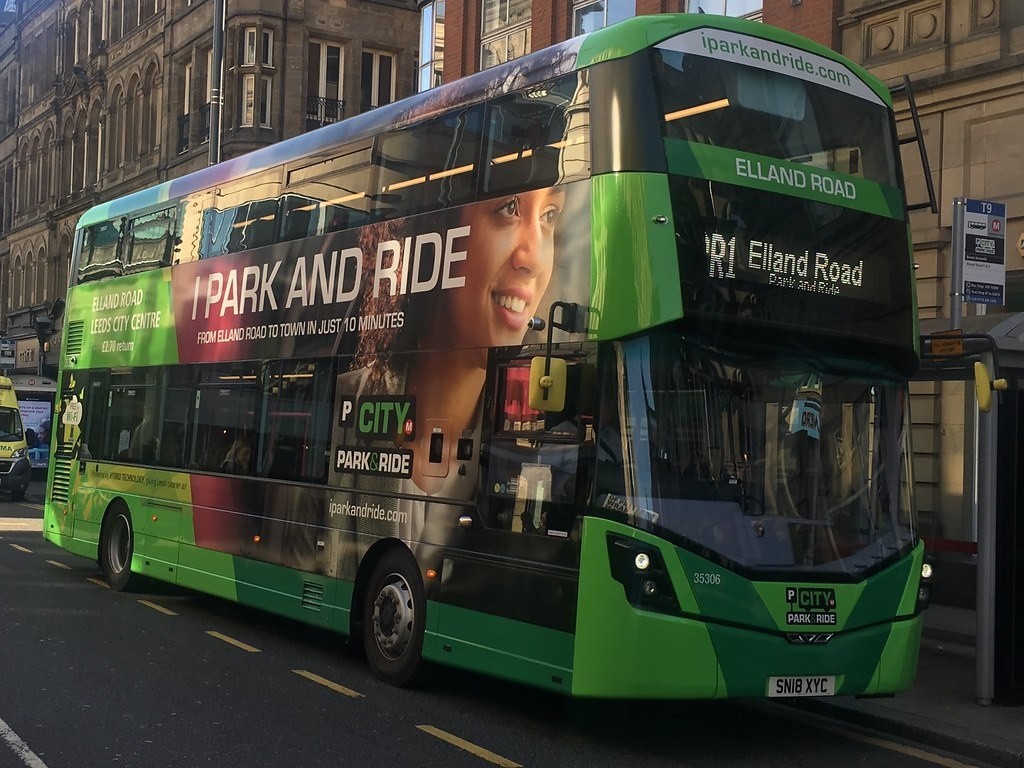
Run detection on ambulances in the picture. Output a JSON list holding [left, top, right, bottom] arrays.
[[0, 374, 36, 504]]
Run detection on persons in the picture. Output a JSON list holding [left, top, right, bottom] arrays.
[[538, 387, 624, 518], [216, 440, 268, 477], [37, 421, 52, 448], [250, 56, 590, 595]]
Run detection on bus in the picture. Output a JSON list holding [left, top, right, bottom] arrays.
[[42, 11, 1009, 715]]
